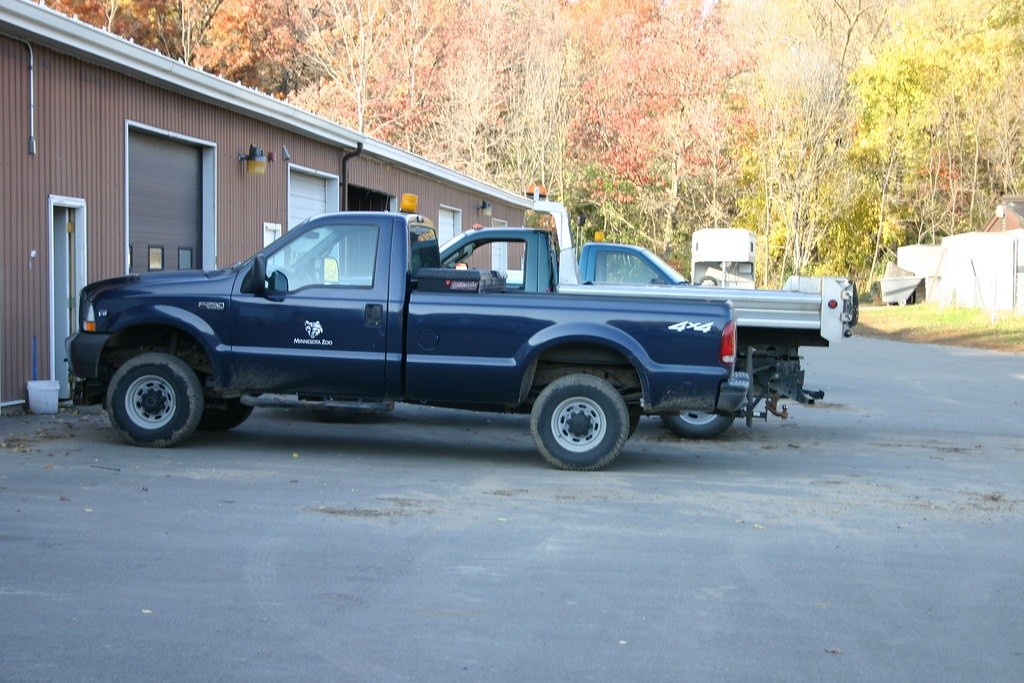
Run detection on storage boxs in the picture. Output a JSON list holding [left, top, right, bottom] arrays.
[[412, 267, 481, 293]]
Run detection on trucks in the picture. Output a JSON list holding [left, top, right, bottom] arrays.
[[687, 228, 758, 292]]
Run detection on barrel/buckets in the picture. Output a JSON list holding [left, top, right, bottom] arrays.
[[27, 380, 60, 414]]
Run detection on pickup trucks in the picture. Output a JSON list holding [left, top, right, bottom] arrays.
[[59, 193, 752, 474], [338, 224, 860, 438]]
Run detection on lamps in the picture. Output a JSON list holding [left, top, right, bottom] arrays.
[[238, 143, 266, 175], [282, 145, 291, 160], [996, 205, 1005, 219], [477, 200, 492, 216]]
